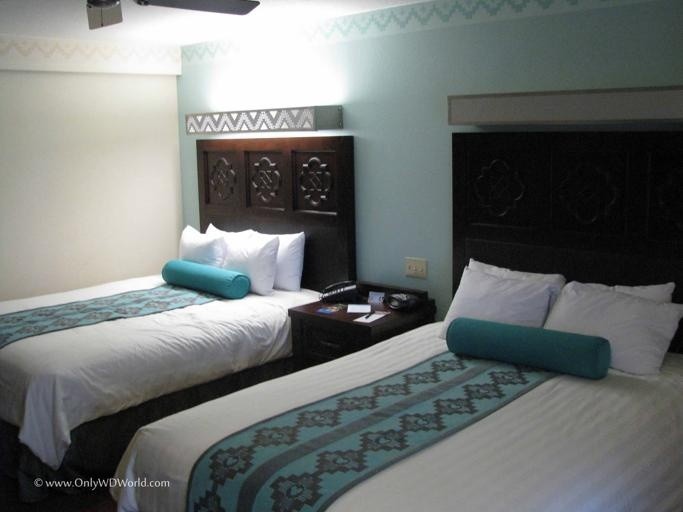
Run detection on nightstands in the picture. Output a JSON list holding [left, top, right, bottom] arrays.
[[288, 282, 438, 373]]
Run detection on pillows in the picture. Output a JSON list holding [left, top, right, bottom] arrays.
[[254, 232, 306, 292], [544, 281, 683, 376], [446, 317, 610, 381], [204, 221, 252, 235], [179, 224, 225, 267], [468, 256, 566, 316], [223, 234, 279, 295], [438, 267, 555, 341], [162, 259, 250, 299], [585, 282, 675, 304]]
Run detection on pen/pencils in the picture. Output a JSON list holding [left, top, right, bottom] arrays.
[[365, 310, 375, 319]]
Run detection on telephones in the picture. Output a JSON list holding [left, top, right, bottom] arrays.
[[319, 280, 361, 301]]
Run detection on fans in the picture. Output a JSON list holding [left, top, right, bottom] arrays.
[[86, 0, 260, 30]]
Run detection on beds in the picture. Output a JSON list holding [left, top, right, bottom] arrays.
[[0, 272, 324, 472], [107, 320, 683, 512]]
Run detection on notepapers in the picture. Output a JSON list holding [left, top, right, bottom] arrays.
[[347, 304, 371, 313]]
